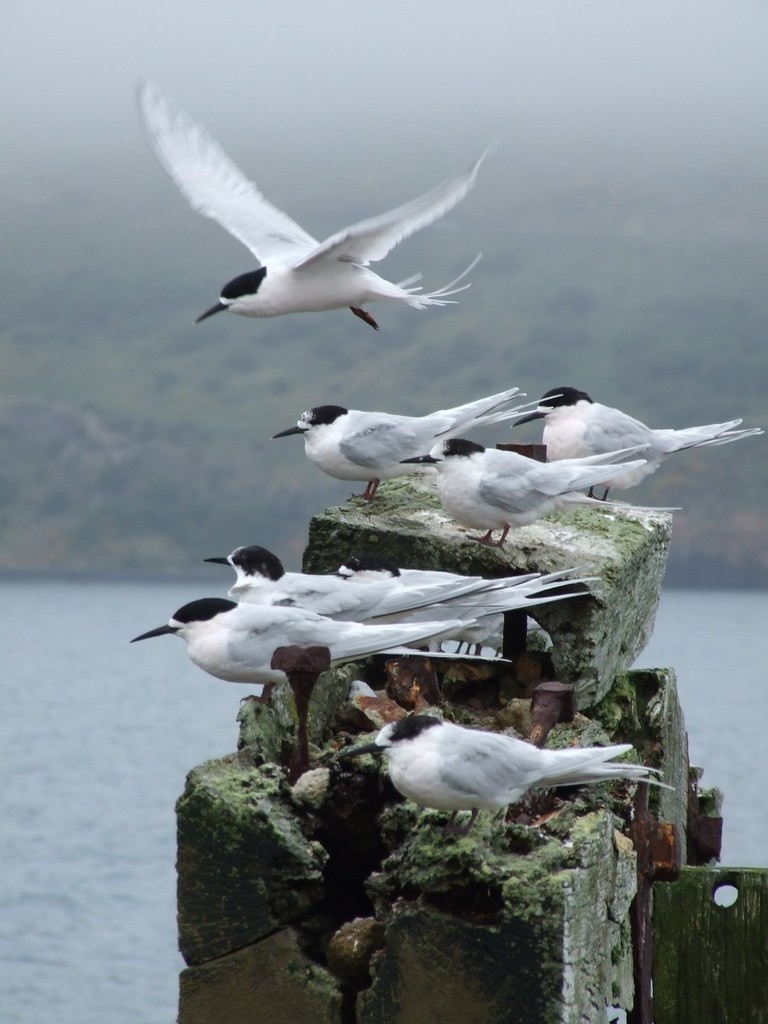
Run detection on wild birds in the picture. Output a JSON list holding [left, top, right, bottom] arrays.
[[400, 440, 649, 550], [512, 386, 764, 501], [336, 714, 676, 839], [129, 545, 601, 782], [138, 80, 488, 331], [270, 388, 562, 499]]
